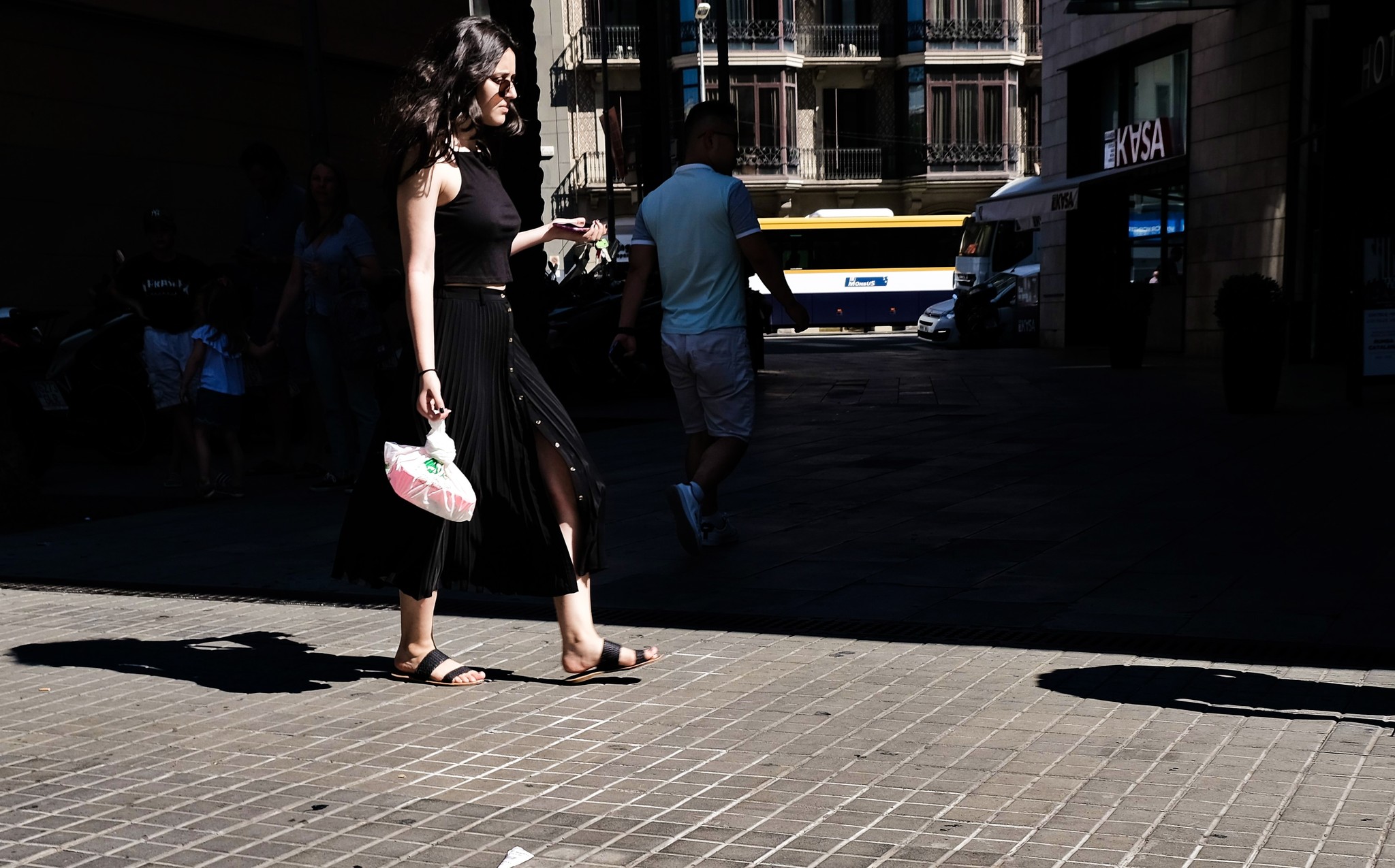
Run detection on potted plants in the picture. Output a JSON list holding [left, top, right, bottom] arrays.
[[1108, 280, 1155, 369], [1214, 274, 1285, 415]]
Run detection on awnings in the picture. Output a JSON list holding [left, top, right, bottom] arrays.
[[976, 153, 1189, 223]]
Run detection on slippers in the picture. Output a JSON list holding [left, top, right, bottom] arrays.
[[564, 639, 663, 685], [390, 648, 484, 687]]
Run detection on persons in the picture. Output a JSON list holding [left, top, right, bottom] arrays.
[[109, 141, 381, 499], [386, 15, 657, 688], [609, 99, 811, 554]]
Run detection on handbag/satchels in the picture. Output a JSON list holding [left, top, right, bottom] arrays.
[[384, 410, 476, 522]]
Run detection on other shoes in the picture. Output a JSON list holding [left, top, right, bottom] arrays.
[[308, 473, 353, 494], [163, 473, 198, 488], [194, 480, 246, 500], [670, 480, 707, 546]]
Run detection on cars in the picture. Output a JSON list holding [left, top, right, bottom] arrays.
[[917, 263, 1041, 346]]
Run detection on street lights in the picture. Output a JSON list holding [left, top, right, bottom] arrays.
[[695, 0, 712, 102]]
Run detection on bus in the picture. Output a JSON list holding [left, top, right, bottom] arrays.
[[952, 199, 1185, 285], [548, 207, 974, 335]]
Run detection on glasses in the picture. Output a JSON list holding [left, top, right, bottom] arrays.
[[489, 76, 519, 98]]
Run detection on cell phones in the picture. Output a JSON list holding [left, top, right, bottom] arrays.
[[552, 221, 593, 232], [609, 339, 637, 378]]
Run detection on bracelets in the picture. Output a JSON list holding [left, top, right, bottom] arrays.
[[616, 326, 637, 337], [418, 369, 438, 377]]
